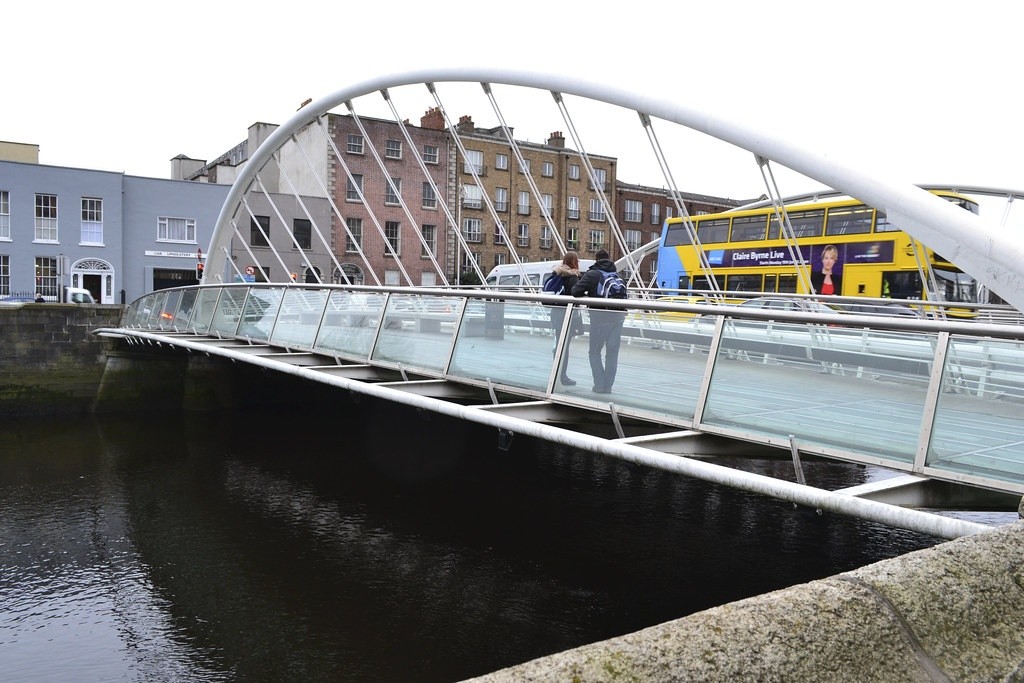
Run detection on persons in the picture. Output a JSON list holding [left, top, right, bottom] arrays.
[[35, 293, 45, 303], [572, 250, 628, 393], [809, 245, 843, 296], [542, 252, 584, 386]]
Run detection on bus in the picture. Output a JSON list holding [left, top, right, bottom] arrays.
[[656, 189, 980, 318]]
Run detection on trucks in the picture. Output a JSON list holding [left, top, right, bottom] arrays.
[[45, 287, 97, 304]]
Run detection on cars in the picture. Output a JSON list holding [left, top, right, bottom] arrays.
[[1, 297, 37, 302], [688, 296, 930, 335], [626, 295, 746, 322]]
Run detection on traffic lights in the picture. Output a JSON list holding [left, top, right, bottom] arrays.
[[290, 272, 297, 283], [196, 263, 204, 280]]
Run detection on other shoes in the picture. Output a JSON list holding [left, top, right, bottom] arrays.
[[592, 385, 612, 394], [561, 376, 576, 386]]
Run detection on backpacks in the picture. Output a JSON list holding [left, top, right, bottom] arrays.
[[587, 269, 627, 310], [542, 274, 566, 306]]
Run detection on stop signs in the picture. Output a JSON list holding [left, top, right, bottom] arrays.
[[198, 248, 201, 260]]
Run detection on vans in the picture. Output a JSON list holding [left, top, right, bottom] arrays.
[[462, 259, 597, 316]]
